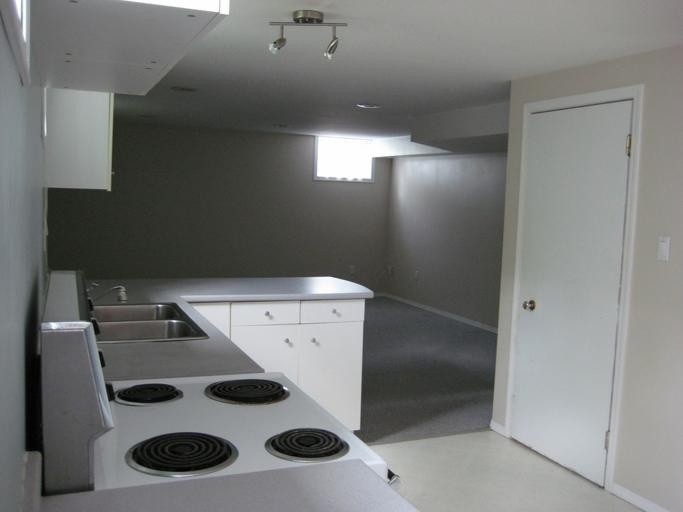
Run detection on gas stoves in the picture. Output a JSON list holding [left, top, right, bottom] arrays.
[[97, 370, 387, 489]]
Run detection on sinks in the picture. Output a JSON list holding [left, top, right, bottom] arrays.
[[91, 303, 184, 321], [97, 320, 207, 343]]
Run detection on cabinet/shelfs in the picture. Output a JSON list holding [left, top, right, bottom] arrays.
[[186, 298, 366, 434]]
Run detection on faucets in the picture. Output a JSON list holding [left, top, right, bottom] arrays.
[[93, 285, 128, 306]]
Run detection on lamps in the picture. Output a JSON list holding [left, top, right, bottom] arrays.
[[268, 10, 348, 61]]
